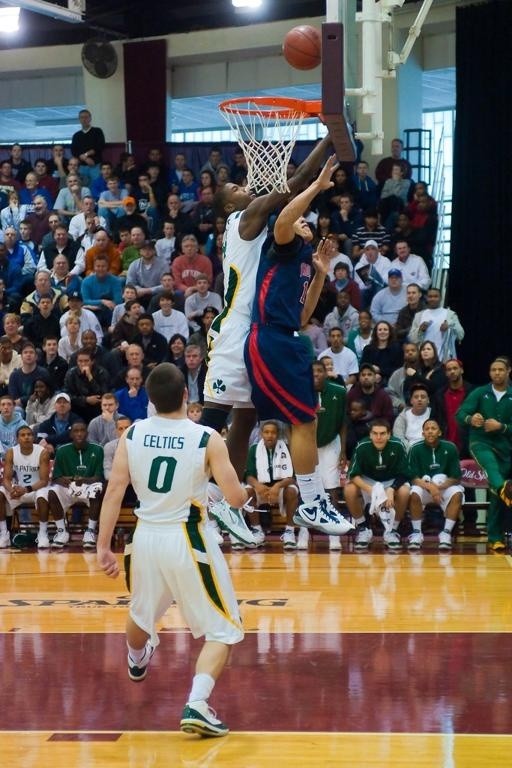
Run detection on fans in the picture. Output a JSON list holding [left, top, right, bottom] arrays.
[[78, 32, 118, 79]]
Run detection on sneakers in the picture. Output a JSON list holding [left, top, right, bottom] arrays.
[[383, 529, 406, 550], [0, 523, 95, 550], [486, 536, 507, 552], [406, 526, 427, 550], [500, 478, 512, 508], [126, 636, 157, 683], [293, 491, 358, 537], [353, 527, 376, 551], [179, 700, 232, 738], [435, 531, 454, 549], [205, 494, 257, 549], [226, 524, 343, 551]]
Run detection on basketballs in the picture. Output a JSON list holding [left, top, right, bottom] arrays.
[[281, 24, 322, 70]]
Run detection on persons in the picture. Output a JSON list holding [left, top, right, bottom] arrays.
[[374, 138, 413, 180], [70, 109, 106, 178], [352, 127, 365, 164], [96, 362, 251, 737]]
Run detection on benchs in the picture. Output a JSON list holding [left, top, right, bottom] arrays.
[[1, 498, 494, 553]]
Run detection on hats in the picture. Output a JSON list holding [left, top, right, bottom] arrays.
[[359, 362, 375, 373], [138, 238, 157, 250], [364, 239, 379, 251], [123, 195, 137, 207], [68, 290, 84, 302], [54, 393, 72, 404], [388, 267, 403, 277]]
